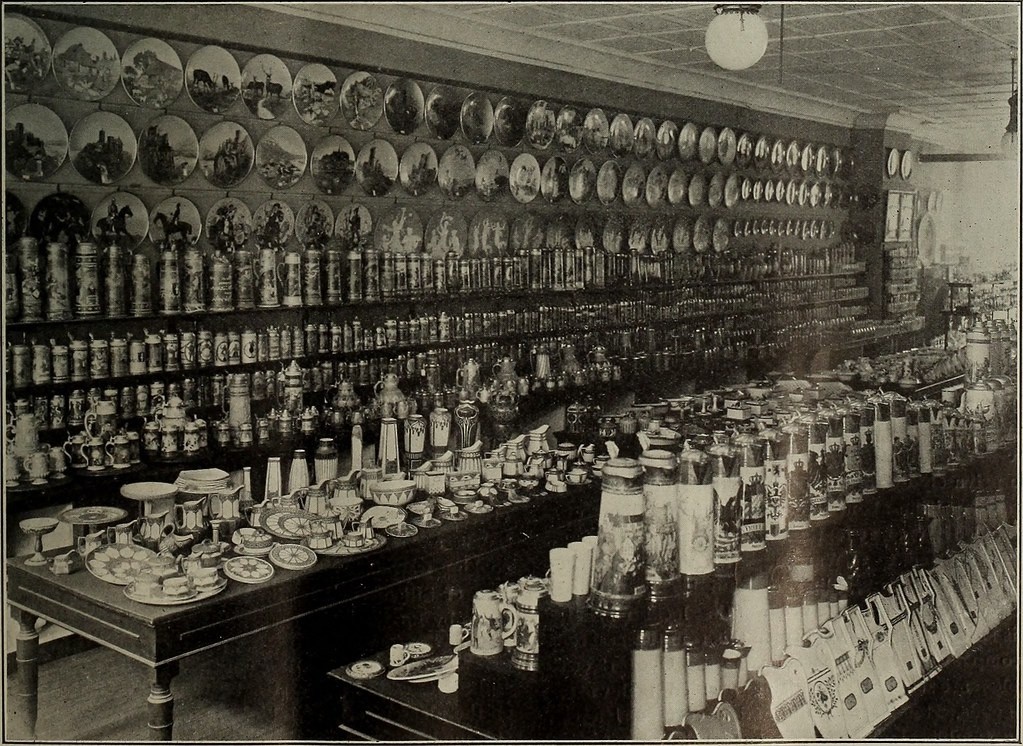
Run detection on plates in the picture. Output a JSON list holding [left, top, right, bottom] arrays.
[[403, 642, 431, 655], [4, 14, 944, 261], [388, 652, 459, 682], [12, 461, 493, 611], [344, 658, 386, 679]]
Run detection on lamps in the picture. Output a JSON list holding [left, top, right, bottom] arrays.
[[998, 58, 1019, 160], [703, 2, 770, 71]]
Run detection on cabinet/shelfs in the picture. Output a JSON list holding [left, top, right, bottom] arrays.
[[3, 241, 887, 512]]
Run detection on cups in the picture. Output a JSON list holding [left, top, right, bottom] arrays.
[[449, 313, 1015, 745], [4, 238, 898, 488], [389, 643, 410, 666]]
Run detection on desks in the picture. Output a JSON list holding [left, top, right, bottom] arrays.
[[326, 443, 1020, 743], [3, 448, 615, 743]]
